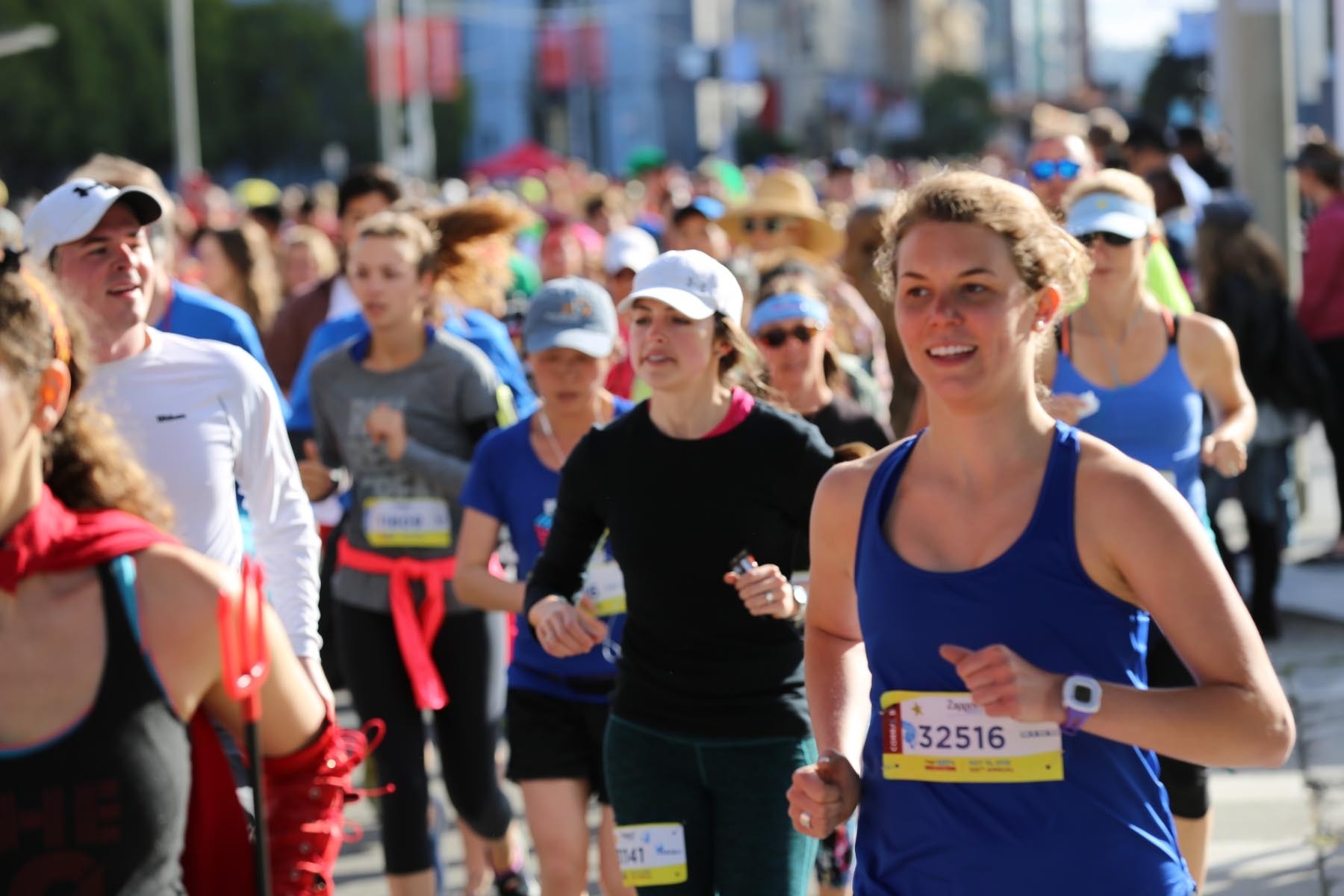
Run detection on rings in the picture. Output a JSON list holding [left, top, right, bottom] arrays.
[[800, 812, 811, 827]]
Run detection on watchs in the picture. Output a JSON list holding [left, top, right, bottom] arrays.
[[788, 585, 807, 621], [1059, 672, 1103, 736]]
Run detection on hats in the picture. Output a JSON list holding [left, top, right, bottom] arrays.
[[25, 176, 162, 266], [1201, 196, 1255, 231], [1063, 191, 1156, 239], [618, 248, 743, 332], [1283, 153, 1319, 172], [604, 224, 659, 275], [670, 195, 724, 221], [717, 169, 837, 259], [233, 178, 283, 209], [521, 276, 617, 358]]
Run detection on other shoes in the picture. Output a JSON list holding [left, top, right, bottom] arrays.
[[486, 841, 527, 896]]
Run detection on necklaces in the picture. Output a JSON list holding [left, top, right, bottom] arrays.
[[537, 397, 569, 464], [163, 281, 174, 329]]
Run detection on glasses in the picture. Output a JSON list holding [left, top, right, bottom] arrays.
[[1023, 158, 1081, 180], [756, 325, 821, 349], [739, 217, 799, 235], [1075, 232, 1134, 247]]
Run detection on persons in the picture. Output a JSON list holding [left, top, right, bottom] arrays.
[[0, 109, 1344, 896], [524, 249, 834, 896], [285, 212, 525, 896], [1034, 168, 1258, 895], [22, 176, 335, 721], [787, 168, 1298, 896], [0, 247, 394, 896], [455, 277, 633, 896]]
